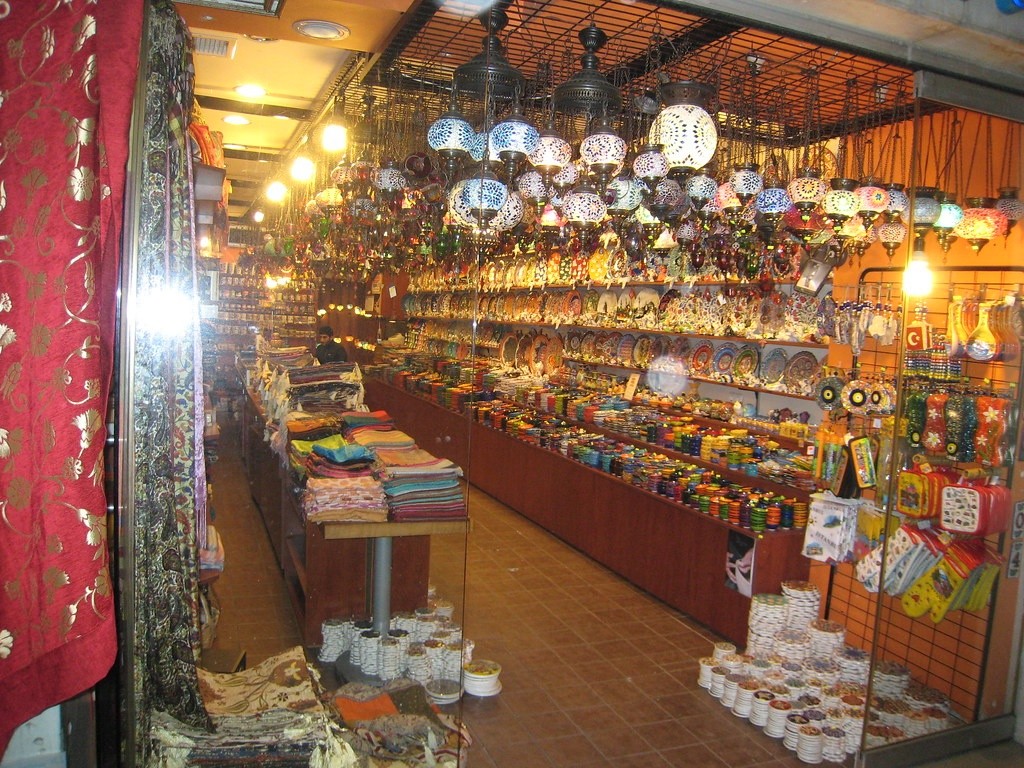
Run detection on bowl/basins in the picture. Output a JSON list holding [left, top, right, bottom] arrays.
[[371, 345, 825, 532]]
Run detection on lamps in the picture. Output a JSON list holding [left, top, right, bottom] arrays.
[[292, 18, 351, 42]]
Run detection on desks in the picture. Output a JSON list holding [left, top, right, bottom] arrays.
[[318, 518, 475, 685]]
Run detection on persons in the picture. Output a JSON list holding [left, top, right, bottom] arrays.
[[314, 326, 347, 365]]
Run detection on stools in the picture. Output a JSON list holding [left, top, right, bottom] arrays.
[[201, 648, 247, 675]]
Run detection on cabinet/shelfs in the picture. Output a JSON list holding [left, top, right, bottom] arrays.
[[201, 85, 1024, 767]]
